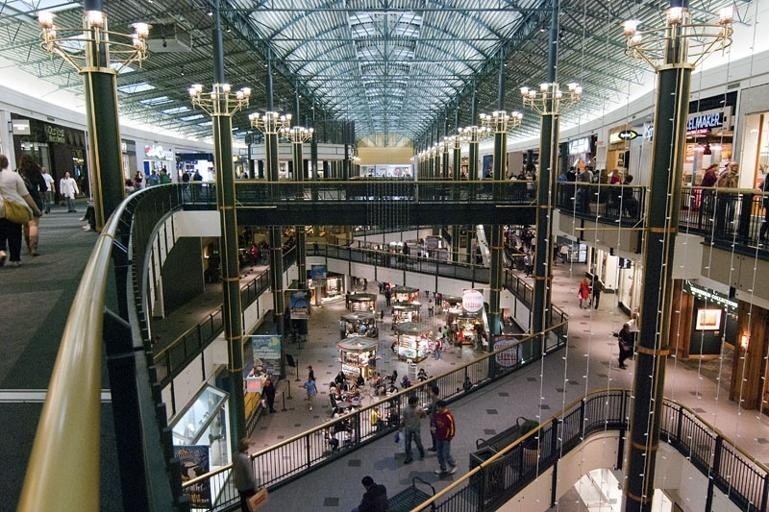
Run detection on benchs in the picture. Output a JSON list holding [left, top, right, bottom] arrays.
[[476, 416, 544, 468], [385, 476, 436, 511]]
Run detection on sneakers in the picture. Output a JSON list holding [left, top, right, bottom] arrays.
[[432, 465, 459, 476], [0, 251, 7, 267], [403, 457, 414, 465]]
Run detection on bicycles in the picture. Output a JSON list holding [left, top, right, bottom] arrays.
[[434, 349, 441, 360]]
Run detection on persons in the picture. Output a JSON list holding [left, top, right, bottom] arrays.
[[441, 169, 537, 201], [553, 242, 640, 369], [313, 225, 537, 276], [351, 475, 387, 512], [232, 436, 260, 512], [260, 278, 484, 453], [240, 169, 324, 181], [560, 165, 639, 219], [1, 154, 96, 265], [430, 403, 461, 475], [125, 167, 216, 194], [701, 162, 769, 242], [398, 396, 427, 464], [248, 232, 296, 267]]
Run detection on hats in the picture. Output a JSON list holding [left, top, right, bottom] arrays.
[[237, 438, 254, 450]]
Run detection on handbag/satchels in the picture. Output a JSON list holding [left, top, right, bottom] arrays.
[[245, 487, 270, 512], [3, 198, 34, 223]]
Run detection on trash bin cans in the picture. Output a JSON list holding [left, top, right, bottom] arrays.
[[470, 446, 505, 499]]
